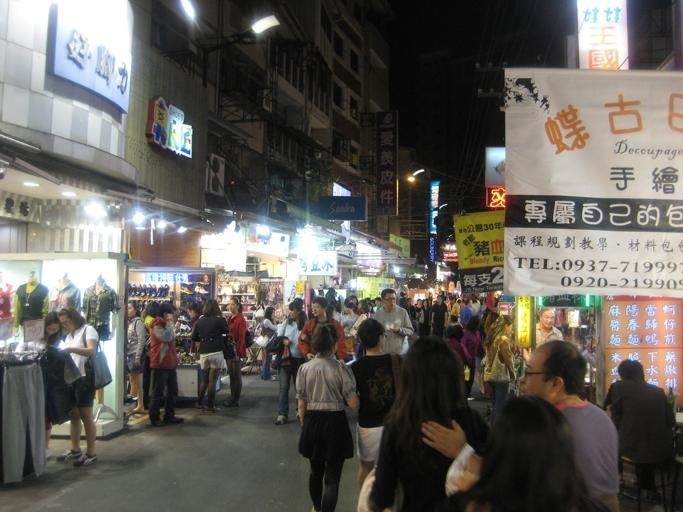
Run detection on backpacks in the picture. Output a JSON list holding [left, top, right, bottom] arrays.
[[133, 318, 153, 355]]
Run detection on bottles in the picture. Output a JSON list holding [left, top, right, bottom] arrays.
[[463, 365, 470, 382]]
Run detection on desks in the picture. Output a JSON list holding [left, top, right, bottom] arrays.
[[245, 347, 263, 377]]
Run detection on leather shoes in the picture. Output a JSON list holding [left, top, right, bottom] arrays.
[[129, 283, 136, 297], [135, 283, 142, 296], [150, 282, 157, 298], [145, 282, 152, 296], [161, 283, 169, 297], [127, 282, 132, 297], [156, 284, 163, 297], [139, 283, 147, 297]]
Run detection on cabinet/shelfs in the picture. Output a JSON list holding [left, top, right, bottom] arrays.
[[217, 293, 256, 328], [133, 357, 223, 402]]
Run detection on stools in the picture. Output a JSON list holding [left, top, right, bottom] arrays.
[[619, 456, 677, 512]]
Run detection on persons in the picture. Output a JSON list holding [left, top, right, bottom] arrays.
[[297, 283, 673, 512], [42, 307, 96, 465], [260, 298, 309, 424], [0, 271, 120, 353], [127, 298, 246, 426]]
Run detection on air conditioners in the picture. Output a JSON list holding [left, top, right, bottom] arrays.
[[205, 152, 226, 198], [260, 89, 273, 113]]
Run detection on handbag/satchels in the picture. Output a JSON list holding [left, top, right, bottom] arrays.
[[255, 331, 275, 348], [481, 335, 519, 385], [222, 334, 235, 360], [418, 310, 425, 324], [259, 326, 274, 338], [82, 352, 113, 393], [244, 329, 254, 348]]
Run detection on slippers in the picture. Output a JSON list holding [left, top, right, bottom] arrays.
[[127, 407, 145, 415]]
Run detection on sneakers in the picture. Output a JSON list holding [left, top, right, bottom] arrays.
[[193, 282, 209, 295], [44, 448, 53, 459], [55, 448, 83, 463], [271, 376, 275, 380], [72, 453, 98, 467], [205, 407, 220, 413], [175, 286, 193, 295], [150, 418, 165, 426], [220, 396, 239, 407], [161, 416, 184, 425], [195, 400, 204, 409], [273, 415, 288, 425]]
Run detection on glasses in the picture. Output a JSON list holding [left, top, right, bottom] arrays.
[[519, 365, 557, 378]]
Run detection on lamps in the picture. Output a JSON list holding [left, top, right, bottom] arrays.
[[177, 11, 282, 61]]
